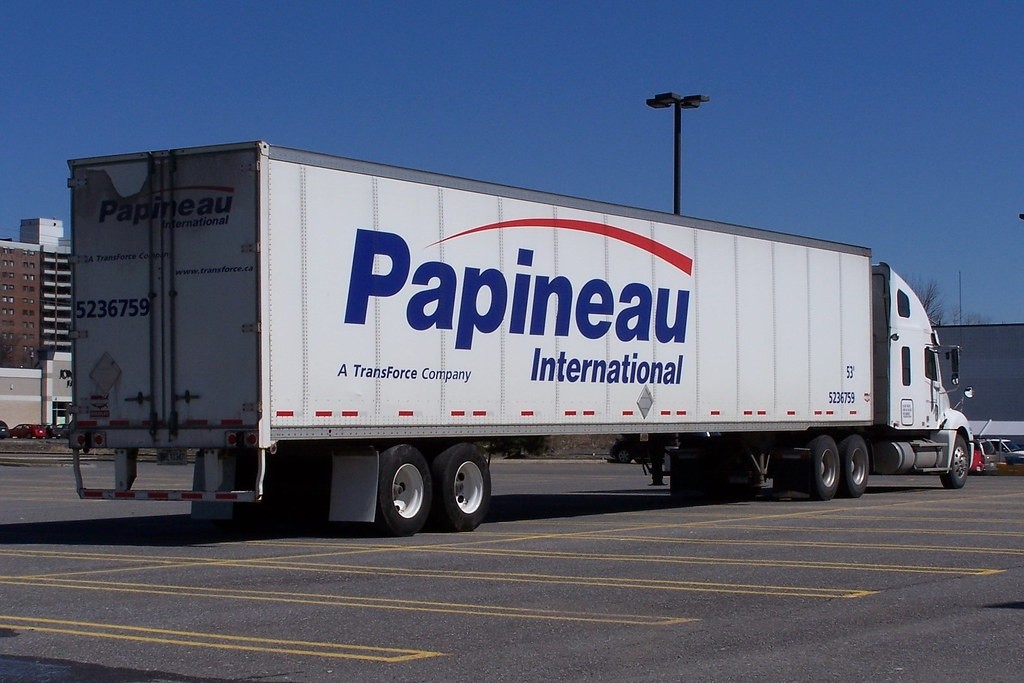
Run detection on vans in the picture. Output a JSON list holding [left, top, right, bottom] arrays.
[[609, 431, 722, 464]]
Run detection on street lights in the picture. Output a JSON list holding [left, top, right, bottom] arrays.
[[646, 92, 711, 214]]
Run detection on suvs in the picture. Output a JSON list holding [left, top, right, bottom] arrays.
[[967, 439, 1024, 475]]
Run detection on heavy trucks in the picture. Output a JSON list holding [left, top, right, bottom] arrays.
[[66, 139, 976, 540]]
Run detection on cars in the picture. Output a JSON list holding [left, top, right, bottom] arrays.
[[0, 420, 70, 439]]
